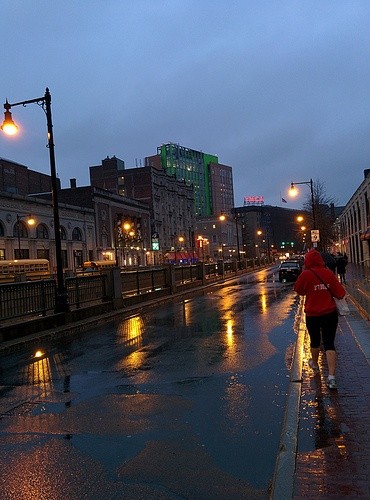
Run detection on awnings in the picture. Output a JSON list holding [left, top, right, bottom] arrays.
[[167, 253, 198, 259]]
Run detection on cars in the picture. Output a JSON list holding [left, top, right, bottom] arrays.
[[277, 255, 305, 282]]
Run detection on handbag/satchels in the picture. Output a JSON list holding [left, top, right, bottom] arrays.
[[334, 294, 350, 316]]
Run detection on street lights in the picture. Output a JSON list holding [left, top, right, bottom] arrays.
[[179, 226, 192, 262], [16, 213, 34, 259], [257, 228, 270, 256], [288, 178, 317, 251], [219, 209, 240, 262], [1, 87, 70, 314], [113, 220, 135, 266]]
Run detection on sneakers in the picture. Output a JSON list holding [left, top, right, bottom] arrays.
[[309, 358, 320, 374], [326, 377, 337, 388]]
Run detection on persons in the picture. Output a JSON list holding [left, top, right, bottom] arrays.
[[294, 250, 346, 388], [322, 252, 348, 285]]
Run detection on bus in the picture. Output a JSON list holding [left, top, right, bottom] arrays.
[[0, 258, 51, 284], [81, 260, 116, 271]]
